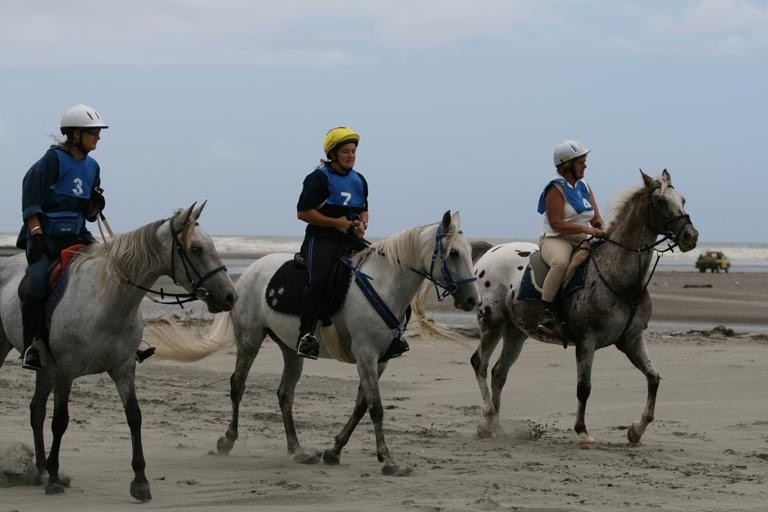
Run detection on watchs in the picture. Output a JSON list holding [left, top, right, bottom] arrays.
[[362, 219, 368, 230]]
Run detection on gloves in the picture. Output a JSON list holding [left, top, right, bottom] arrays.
[[91, 189, 105, 212], [26, 233, 45, 266]]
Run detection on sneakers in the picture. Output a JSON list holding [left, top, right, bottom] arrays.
[[394, 340, 409, 354], [21, 344, 41, 366], [135, 347, 156, 363], [537, 312, 554, 328], [296, 336, 320, 356]]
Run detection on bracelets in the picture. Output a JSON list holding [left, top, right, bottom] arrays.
[[31, 226, 40, 234]]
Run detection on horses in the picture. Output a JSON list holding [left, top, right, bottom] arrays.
[[0, 200, 239, 503], [410, 169, 702, 450], [143, 209, 481, 476]]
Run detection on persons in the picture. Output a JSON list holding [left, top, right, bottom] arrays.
[[537, 141, 607, 333], [297, 127, 410, 356], [15, 105, 156, 371]]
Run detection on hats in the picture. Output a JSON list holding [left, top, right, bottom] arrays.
[[60, 104, 109, 129], [554, 140, 592, 165], [323, 127, 359, 155]]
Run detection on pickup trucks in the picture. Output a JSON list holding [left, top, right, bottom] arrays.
[[696, 251, 731, 274]]
